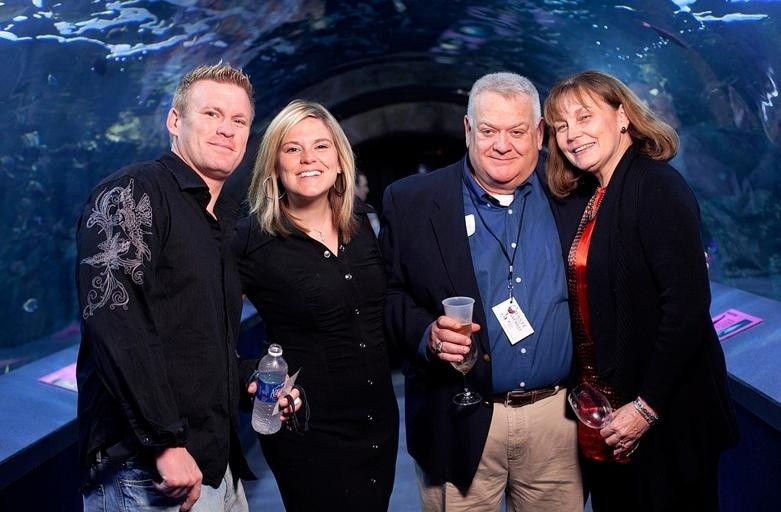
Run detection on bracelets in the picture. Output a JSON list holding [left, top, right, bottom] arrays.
[[633, 398, 659, 426]]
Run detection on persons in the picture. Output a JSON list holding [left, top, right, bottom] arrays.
[[75, 59, 254, 511], [543, 71, 741, 510], [353, 167, 382, 240], [230, 100, 402, 512], [377, 71, 600, 511]]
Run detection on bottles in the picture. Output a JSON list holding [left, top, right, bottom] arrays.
[[251, 343, 288, 435]]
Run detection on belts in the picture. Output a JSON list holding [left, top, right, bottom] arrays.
[[494, 384, 565, 407]]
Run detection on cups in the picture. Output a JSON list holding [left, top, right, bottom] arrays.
[[441, 296, 475, 338]]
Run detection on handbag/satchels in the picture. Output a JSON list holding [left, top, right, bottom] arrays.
[[576, 371, 633, 466]]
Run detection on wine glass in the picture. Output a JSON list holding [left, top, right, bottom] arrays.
[[450, 342, 483, 407], [566, 383, 640, 457]]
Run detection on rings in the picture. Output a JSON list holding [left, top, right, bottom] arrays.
[[620, 441, 625, 448], [436, 340, 444, 353]]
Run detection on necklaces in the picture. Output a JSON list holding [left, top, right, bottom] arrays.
[[587, 185, 606, 222], [302, 218, 328, 241]]
